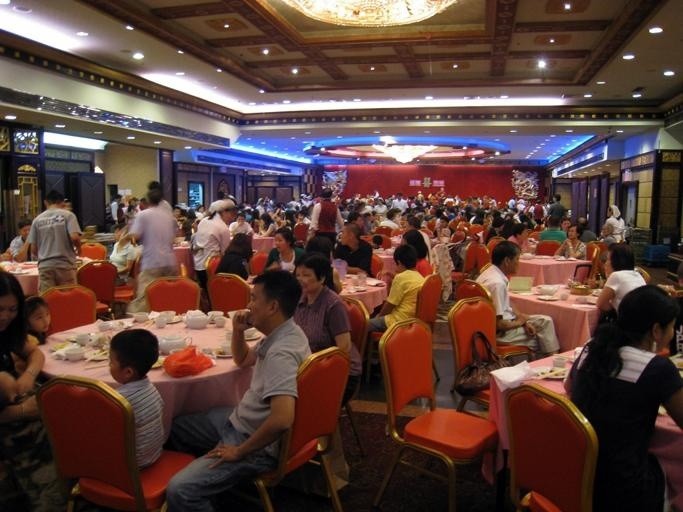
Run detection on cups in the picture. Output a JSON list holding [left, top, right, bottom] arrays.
[[553, 357, 566, 369]]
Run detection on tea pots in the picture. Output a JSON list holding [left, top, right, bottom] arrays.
[[158, 335, 192, 354], [536, 283, 559, 296], [181, 311, 213, 329]]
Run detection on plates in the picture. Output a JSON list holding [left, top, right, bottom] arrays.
[[215, 350, 232, 358], [538, 368, 566, 379], [244, 334, 261, 340], [167, 317, 181, 323]]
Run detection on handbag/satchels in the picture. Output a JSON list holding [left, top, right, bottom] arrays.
[[454, 331, 532, 397]]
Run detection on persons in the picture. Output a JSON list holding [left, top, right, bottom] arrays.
[[597, 244, 646, 311], [474, 238, 560, 358], [41, 329, 168, 511], [164, 271, 315, 512], [289, 251, 361, 410], [1, 269, 69, 510], [1, 181, 627, 325], [567, 285, 683, 512]]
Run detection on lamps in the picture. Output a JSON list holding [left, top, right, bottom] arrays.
[[282, 0, 457, 30]]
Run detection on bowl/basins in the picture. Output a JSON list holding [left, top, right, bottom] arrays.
[[75, 335, 88, 346], [133, 312, 146, 323], [66, 350, 84, 362], [213, 317, 225, 328], [208, 310, 224, 324]]
